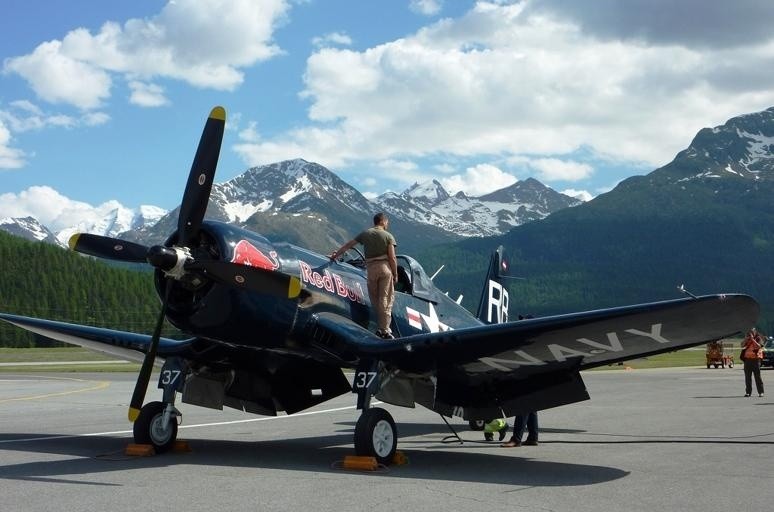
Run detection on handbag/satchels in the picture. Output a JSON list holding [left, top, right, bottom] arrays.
[[740, 349, 746, 361]]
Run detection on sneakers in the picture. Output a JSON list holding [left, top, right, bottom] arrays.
[[759, 393, 764, 397], [498, 422, 509, 440], [744, 393, 751, 397], [376, 329, 395, 339], [485, 432, 494, 441]]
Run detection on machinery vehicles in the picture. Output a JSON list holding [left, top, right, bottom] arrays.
[[706, 338, 735, 368]]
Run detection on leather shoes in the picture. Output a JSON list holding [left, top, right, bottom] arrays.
[[522, 440, 539, 446], [500, 439, 522, 448]]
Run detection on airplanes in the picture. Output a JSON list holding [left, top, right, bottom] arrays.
[[0, 103, 764, 474]]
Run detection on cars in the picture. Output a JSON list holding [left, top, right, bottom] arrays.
[[759, 334, 774, 369]]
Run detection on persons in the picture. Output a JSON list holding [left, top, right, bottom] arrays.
[[482, 418, 508, 441], [324, 213, 399, 339], [500, 314, 540, 447], [739, 327, 765, 397]]
